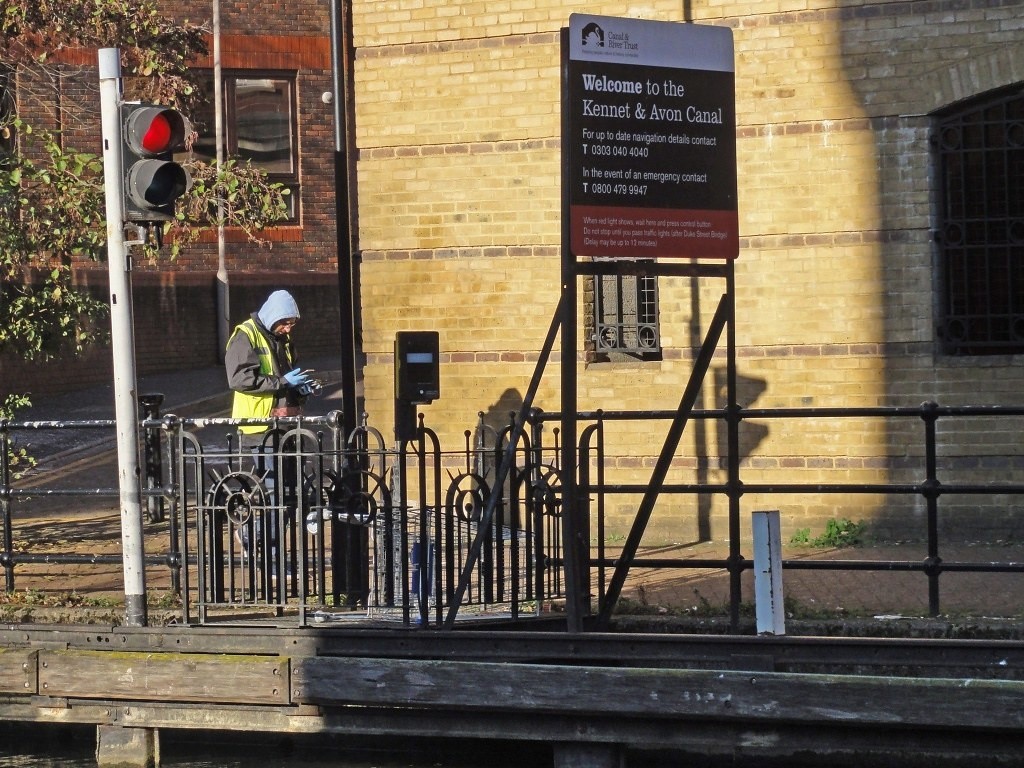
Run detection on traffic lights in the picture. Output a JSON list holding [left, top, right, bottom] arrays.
[[117, 102, 189, 226]]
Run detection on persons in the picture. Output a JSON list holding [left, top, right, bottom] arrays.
[[225, 289, 321, 579]]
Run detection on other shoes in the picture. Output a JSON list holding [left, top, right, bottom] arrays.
[[271, 565, 300, 580], [237, 526, 259, 560]]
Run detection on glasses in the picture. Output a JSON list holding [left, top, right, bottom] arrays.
[[279, 320, 297, 326]]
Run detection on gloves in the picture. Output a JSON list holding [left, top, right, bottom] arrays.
[[298, 384, 312, 395], [284, 369, 310, 387]]
